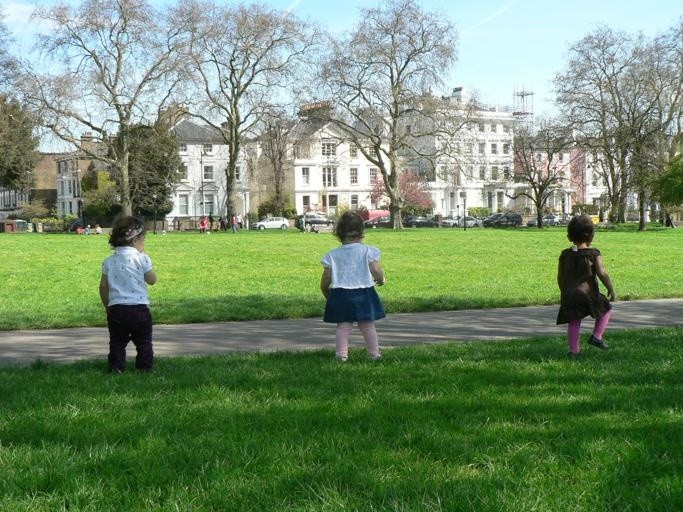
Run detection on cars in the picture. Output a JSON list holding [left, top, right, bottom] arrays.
[[251, 216, 291, 230], [444, 215, 483, 229], [366, 214, 407, 228], [482, 211, 523, 228], [527, 215, 561, 227], [295, 211, 333, 232], [403, 215, 439, 229], [77, 223, 101, 235]]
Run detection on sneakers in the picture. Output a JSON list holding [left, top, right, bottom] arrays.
[[367, 352, 383, 360], [588, 335, 608, 349], [568, 351, 581, 357], [335, 355, 348, 362]]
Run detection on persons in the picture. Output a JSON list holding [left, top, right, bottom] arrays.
[[200, 216, 205, 233], [319, 210, 387, 363], [76, 225, 84, 235], [554, 213, 616, 358], [231, 215, 239, 233], [209, 212, 213, 231], [219, 217, 227, 232], [95, 223, 103, 235], [85, 224, 92, 236], [95, 215, 158, 373]]
